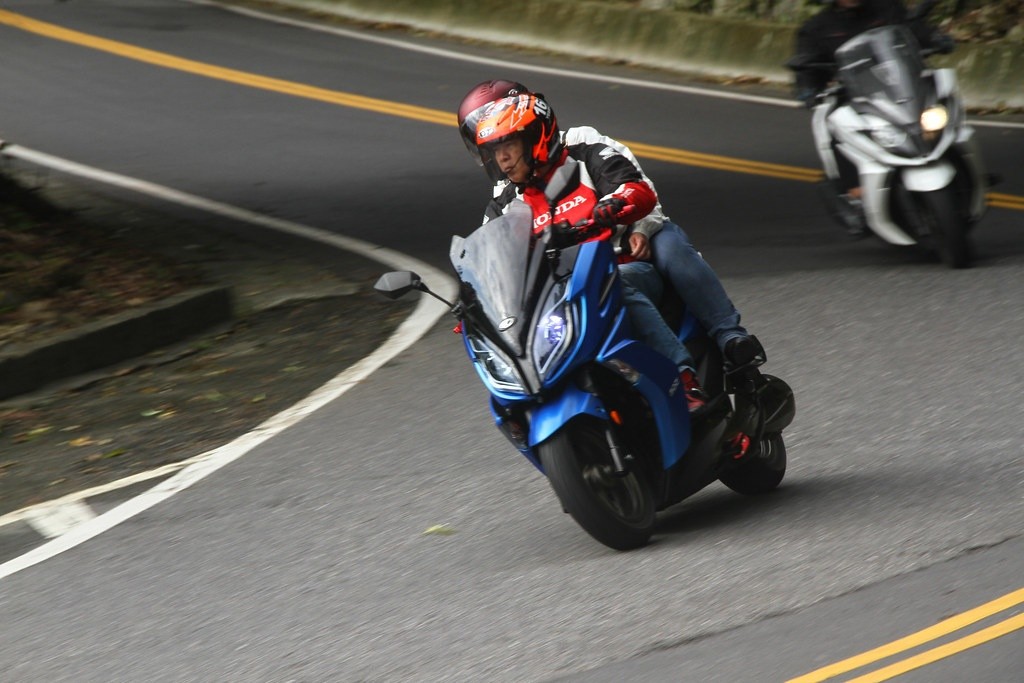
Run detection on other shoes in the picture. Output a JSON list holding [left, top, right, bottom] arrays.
[[842, 197, 866, 233], [984, 168, 1003, 186]]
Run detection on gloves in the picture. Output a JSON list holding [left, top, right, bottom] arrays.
[[799, 86, 823, 108], [929, 33, 953, 54], [592, 198, 629, 228]]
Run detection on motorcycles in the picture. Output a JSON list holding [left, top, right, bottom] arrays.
[[782, 0, 996, 268], [373, 156, 795, 551]]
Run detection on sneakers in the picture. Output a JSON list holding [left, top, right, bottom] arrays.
[[724, 337, 756, 365], [682, 370, 708, 413]]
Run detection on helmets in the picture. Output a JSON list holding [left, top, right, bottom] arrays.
[[458, 79, 544, 143], [476, 94, 560, 165]]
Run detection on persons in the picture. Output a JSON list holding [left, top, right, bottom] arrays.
[[791, 0, 955, 210], [460, 79, 763, 411]]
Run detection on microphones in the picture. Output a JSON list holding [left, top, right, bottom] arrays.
[[497, 154, 524, 183]]
